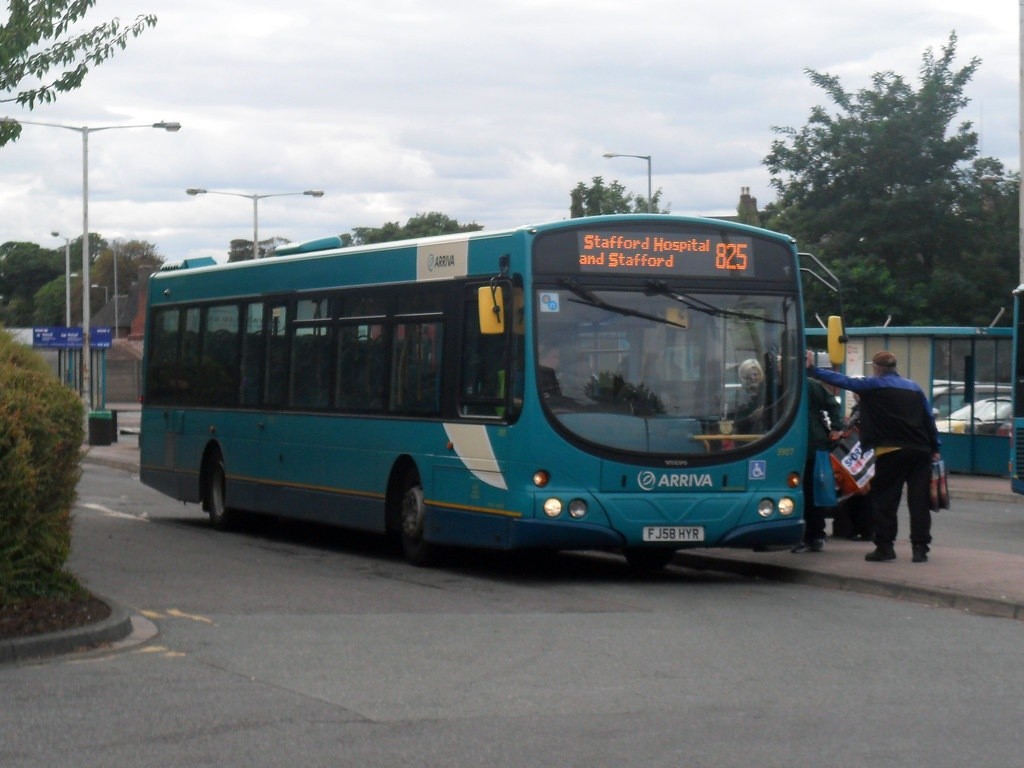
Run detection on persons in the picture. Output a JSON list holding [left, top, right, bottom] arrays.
[[727, 353, 861, 554], [807, 351, 938, 562]]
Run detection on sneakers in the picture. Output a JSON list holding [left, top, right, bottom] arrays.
[[791, 539, 823, 554]]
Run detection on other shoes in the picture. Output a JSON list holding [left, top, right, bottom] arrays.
[[912, 545, 928, 562], [865, 543, 896, 562]]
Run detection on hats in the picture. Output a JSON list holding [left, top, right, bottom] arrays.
[[873, 351, 896, 366]]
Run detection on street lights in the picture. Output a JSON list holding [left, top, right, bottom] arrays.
[[604, 153, 653, 214], [1, 118, 181, 442], [187, 186, 324, 260], [91, 284, 108, 305], [50, 230, 74, 327]]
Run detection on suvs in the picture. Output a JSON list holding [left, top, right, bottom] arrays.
[[933, 384, 1011, 421]]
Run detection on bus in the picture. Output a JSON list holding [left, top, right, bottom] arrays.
[[137, 216, 848, 575]]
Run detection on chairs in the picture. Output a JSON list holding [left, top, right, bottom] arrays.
[[154, 325, 435, 416]]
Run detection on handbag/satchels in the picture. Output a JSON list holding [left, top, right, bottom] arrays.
[[814, 451, 854, 507], [829, 425, 875, 495], [929, 460, 950, 513]]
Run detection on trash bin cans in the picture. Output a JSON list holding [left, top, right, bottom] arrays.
[[87, 409, 113, 446]]
